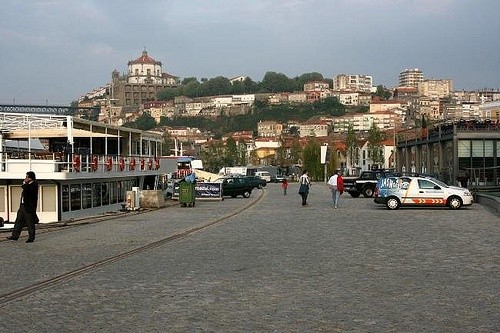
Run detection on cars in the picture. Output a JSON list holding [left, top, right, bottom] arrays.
[[243, 175, 267, 190], [213, 177, 252, 198]]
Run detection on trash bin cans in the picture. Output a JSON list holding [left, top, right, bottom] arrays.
[[178, 182, 197, 206]]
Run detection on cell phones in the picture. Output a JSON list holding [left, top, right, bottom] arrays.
[[29, 178, 32, 180]]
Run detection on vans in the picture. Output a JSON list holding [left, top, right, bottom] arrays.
[[256, 171, 271, 183]]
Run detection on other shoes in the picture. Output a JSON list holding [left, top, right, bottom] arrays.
[[6, 236, 18, 240], [332, 204, 338, 210], [302, 203, 310, 207], [25, 239, 34, 243]]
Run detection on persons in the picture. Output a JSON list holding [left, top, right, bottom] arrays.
[[327, 170, 344, 209], [298, 170, 315, 205], [6, 172, 39, 243], [282, 179, 288, 195]]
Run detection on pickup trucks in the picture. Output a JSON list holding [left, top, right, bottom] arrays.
[[342, 169, 395, 198], [374, 176, 473, 210]]
